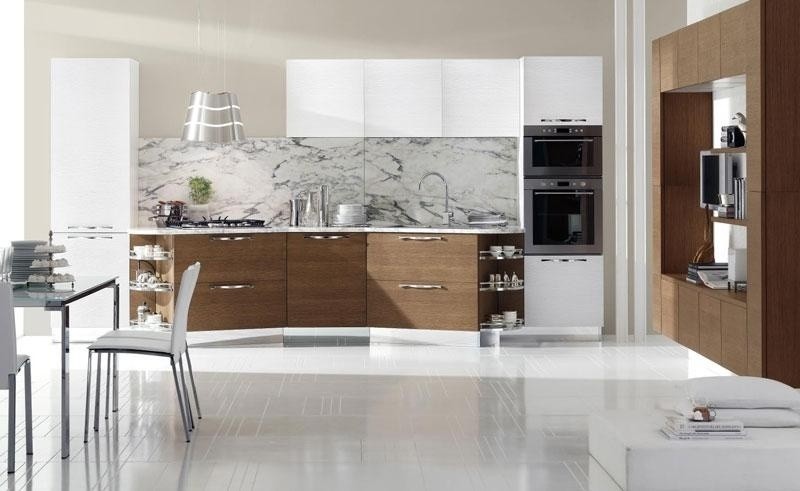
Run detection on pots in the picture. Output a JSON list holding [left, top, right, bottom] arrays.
[[148, 201, 184, 228]]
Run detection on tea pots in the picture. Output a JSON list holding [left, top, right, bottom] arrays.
[[135, 270, 156, 286]]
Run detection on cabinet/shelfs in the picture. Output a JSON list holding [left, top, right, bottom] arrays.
[[659, 33, 677, 93], [286, 58, 363, 138], [480, 234, 526, 333], [699, 16, 720, 85], [369, 234, 480, 346], [363, 59, 444, 140], [721, 303, 749, 375], [522, 256, 603, 342], [445, 58, 522, 135], [523, 56, 603, 124], [129, 232, 174, 334], [720, 1, 800, 76], [675, 26, 699, 94], [172, 236, 287, 333], [47, 56, 139, 339], [287, 233, 368, 331], [680, 284, 700, 352], [661, 278, 679, 341], [700, 297, 722, 371]]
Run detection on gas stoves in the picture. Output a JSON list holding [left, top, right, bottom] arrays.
[[187, 215, 266, 228]]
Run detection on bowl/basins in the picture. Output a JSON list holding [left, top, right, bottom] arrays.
[[503, 311, 517, 326], [153, 245, 163, 257], [503, 244, 515, 256], [133, 245, 152, 258], [489, 245, 502, 256]]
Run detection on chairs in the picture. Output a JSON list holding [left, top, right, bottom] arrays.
[[97, 261, 204, 435], [83, 264, 193, 441], [0, 282, 35, 472]]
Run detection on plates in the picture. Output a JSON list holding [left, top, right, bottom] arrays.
[[10, 240, 51, 283], [466, 213, 505, 230], [335, 204, 366, 226]]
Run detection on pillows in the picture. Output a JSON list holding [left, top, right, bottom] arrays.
[[679, 375, 800, 408], [673, 405, 800, 427]]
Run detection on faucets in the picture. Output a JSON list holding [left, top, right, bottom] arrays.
[[418, 172, 454, 225]]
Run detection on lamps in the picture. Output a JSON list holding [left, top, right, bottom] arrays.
[[181, 0, 248, 145]]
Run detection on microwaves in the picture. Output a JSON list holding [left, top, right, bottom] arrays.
[[525, 128, 605, 256]]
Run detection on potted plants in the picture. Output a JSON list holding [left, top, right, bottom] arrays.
[[189, 177, 213, 222]]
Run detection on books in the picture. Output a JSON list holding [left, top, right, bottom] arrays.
[[659, 416, 748, 440], [734, 177, 747, 220], [685, 262, 729, 290]]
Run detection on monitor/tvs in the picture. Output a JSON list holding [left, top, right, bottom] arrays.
[[699, 151, 733, 212]]
